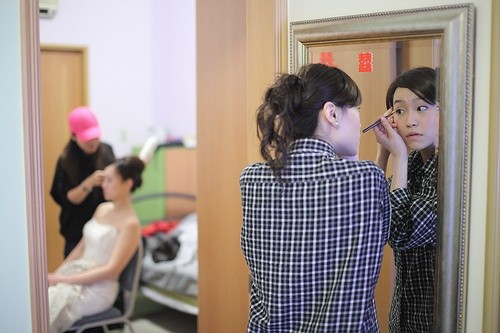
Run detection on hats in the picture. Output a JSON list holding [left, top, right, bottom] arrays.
[[69, 107, 102, 142]]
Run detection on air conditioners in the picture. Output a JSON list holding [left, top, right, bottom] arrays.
[[38, 0, 57, 19]]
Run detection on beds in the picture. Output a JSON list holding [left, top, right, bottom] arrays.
[[132, 146, 199, 316]]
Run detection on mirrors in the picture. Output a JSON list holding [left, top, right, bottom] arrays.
[[291, 2, 475, 333]]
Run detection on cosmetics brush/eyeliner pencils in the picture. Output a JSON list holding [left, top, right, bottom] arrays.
[[362, 111, 395, 133]]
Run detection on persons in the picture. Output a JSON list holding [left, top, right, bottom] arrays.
[[375, 67, 439, 333], [239, 63, 391, 333], [50, 106, 116, 260], [48, 156, 145, 333]]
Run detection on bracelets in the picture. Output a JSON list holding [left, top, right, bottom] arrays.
[[79, 182, 92, 193]]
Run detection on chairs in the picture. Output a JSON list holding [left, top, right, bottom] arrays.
[[65, 239, 144, 333]]
